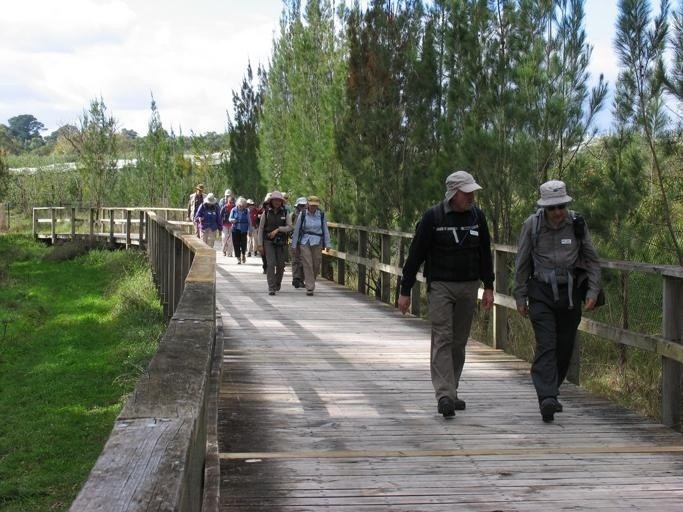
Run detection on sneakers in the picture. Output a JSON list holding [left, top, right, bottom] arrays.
[[456, 396, 465, 410], [299, 282, 305, 287], [306, 291, 313, 296], [555, 399, 563, 412], [540, 399, 556, 422], [437, 397, 456, 417]]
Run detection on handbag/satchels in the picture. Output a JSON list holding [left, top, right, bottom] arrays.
[[573, 210, 606, 309]]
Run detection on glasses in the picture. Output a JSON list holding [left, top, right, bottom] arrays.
[[547, 205, 567, 210]]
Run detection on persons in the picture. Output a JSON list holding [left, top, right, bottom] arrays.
[[184, 181, 332, 296], [395, 167, 495, 417], [512, 181, 603, 422]]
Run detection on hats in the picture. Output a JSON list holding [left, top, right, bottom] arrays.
[[444, 170, 483, 203], [264, 192, 320, 207], [535, 180, 572, 207], [195, 184, 254, 206]]
